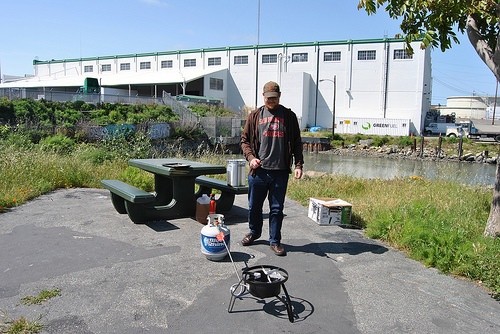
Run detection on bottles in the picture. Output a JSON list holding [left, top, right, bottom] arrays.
[[196, 194, 211, 223], [209, 194, 216, 214]]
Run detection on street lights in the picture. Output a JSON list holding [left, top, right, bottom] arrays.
[[319, 75, 335, 140]]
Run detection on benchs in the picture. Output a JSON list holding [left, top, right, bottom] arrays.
[[194, 175, 250, 196], [99, 179, 157, 205]]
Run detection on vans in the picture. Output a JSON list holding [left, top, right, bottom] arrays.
[[425, 123, 455, 135]]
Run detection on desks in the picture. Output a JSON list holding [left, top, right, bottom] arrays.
[[110, 157, 235, 224]]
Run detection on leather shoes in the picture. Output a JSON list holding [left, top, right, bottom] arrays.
[[270, 245, 285, 255], [241, 234, 262, 246]]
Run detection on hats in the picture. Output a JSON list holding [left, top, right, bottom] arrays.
[[263, 81, 280, 97]]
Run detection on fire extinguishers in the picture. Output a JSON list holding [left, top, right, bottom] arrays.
[[209, 193, 217, 214]]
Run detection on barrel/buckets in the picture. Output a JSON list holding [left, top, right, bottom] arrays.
[[226, 160, 246, 187]]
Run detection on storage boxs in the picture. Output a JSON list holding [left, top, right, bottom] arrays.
[[307, 197, 353, 227]]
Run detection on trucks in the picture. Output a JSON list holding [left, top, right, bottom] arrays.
[[446, 123, 500, 141]]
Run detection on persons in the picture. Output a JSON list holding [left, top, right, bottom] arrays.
[[240, 81, 304, 257]]
